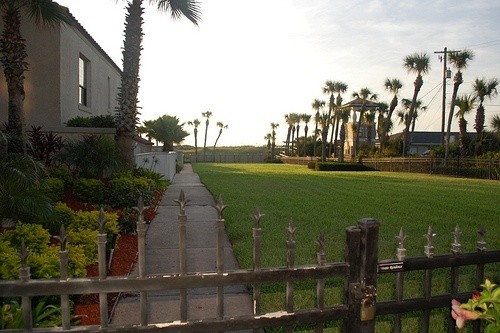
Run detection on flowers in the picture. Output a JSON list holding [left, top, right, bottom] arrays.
[[450, 278, 500, 333]]
